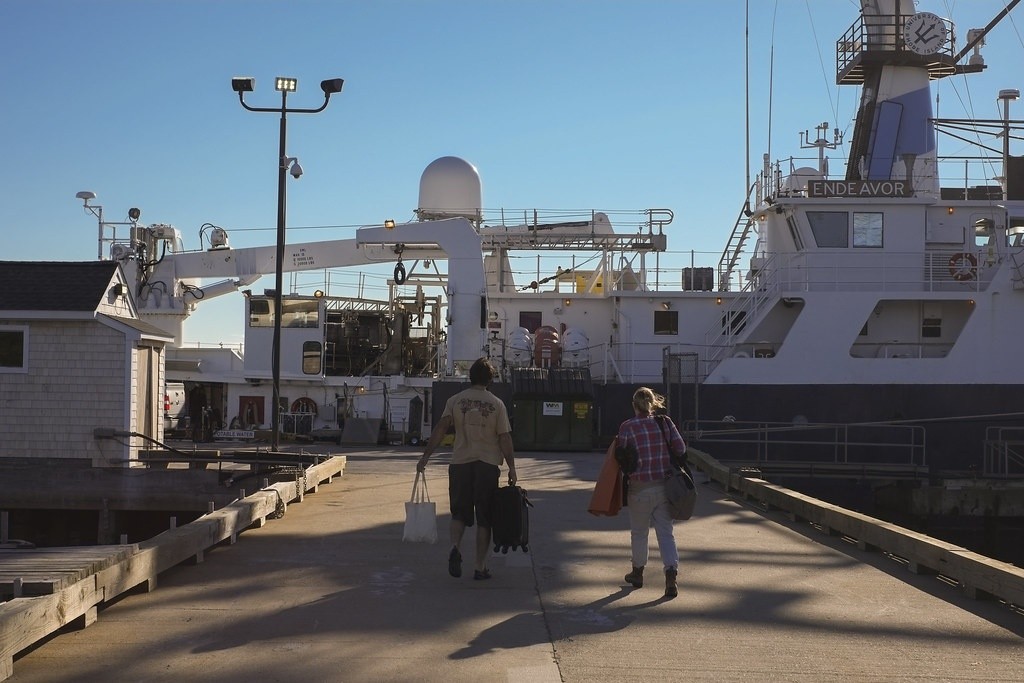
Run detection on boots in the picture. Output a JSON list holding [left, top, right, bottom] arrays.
[[665, 565, 678, 597], [624, 566, 644, 588]]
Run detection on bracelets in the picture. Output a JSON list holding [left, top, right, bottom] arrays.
[[421, 456, 428, 466]]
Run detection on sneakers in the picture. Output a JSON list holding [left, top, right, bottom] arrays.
[[474, 568, 492, 580], [447, 545, 463, 578]]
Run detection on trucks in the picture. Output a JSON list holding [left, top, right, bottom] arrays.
[[164, 382, 188, 434]]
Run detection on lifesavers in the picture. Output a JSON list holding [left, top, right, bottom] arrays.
[[290, 397, 318, 420], [949, 253, 977, 281]]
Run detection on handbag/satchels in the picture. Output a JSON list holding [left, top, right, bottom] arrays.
[[667, 455, 697, 520], [402, 470, 439, 545], [614, 442, 638, 507]]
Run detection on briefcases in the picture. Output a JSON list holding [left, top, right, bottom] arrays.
[[491, 480, 534, 554]]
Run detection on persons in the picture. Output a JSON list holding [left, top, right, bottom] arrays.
[[416, 357, 518, 581], [613, 387, 687, 596]]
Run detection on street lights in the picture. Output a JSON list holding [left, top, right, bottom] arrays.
[[230, 74, 346, 452]]
